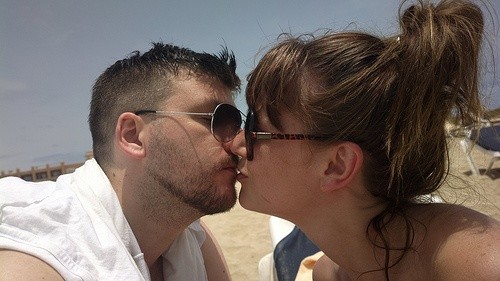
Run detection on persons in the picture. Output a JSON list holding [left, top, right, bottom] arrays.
[[231, 0, 500, 281], [0, 43, 242, 281]]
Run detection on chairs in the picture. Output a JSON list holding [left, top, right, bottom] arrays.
[[258, 195, 441, 281]]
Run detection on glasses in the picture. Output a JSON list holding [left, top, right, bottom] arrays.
[[245, 111, 376, 161], [134, 103, 247, 142]]
